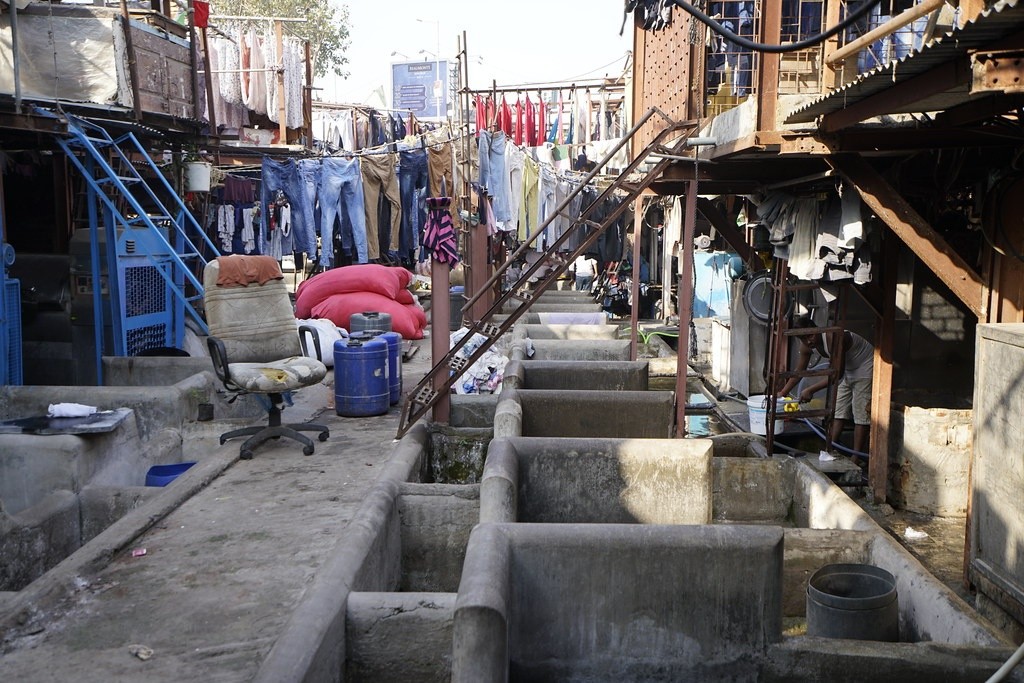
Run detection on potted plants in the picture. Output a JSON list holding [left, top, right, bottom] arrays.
[[161, 144, 212, 200]]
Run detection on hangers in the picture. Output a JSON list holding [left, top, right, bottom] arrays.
[[474, 83, 614, 97]]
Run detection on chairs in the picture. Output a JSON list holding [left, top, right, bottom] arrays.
[[203, 253, 330, 461]]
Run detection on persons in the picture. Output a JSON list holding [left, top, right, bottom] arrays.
[[568, 250, 598, 294], [771, 315, 875, 464]]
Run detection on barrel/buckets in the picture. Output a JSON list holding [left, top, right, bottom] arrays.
[[332, 310, 404, 416], [449, 285, 466, 330], [857, 14, 928, 72], [804, 563, 899, 642], [746, 394, 784, 435]]
